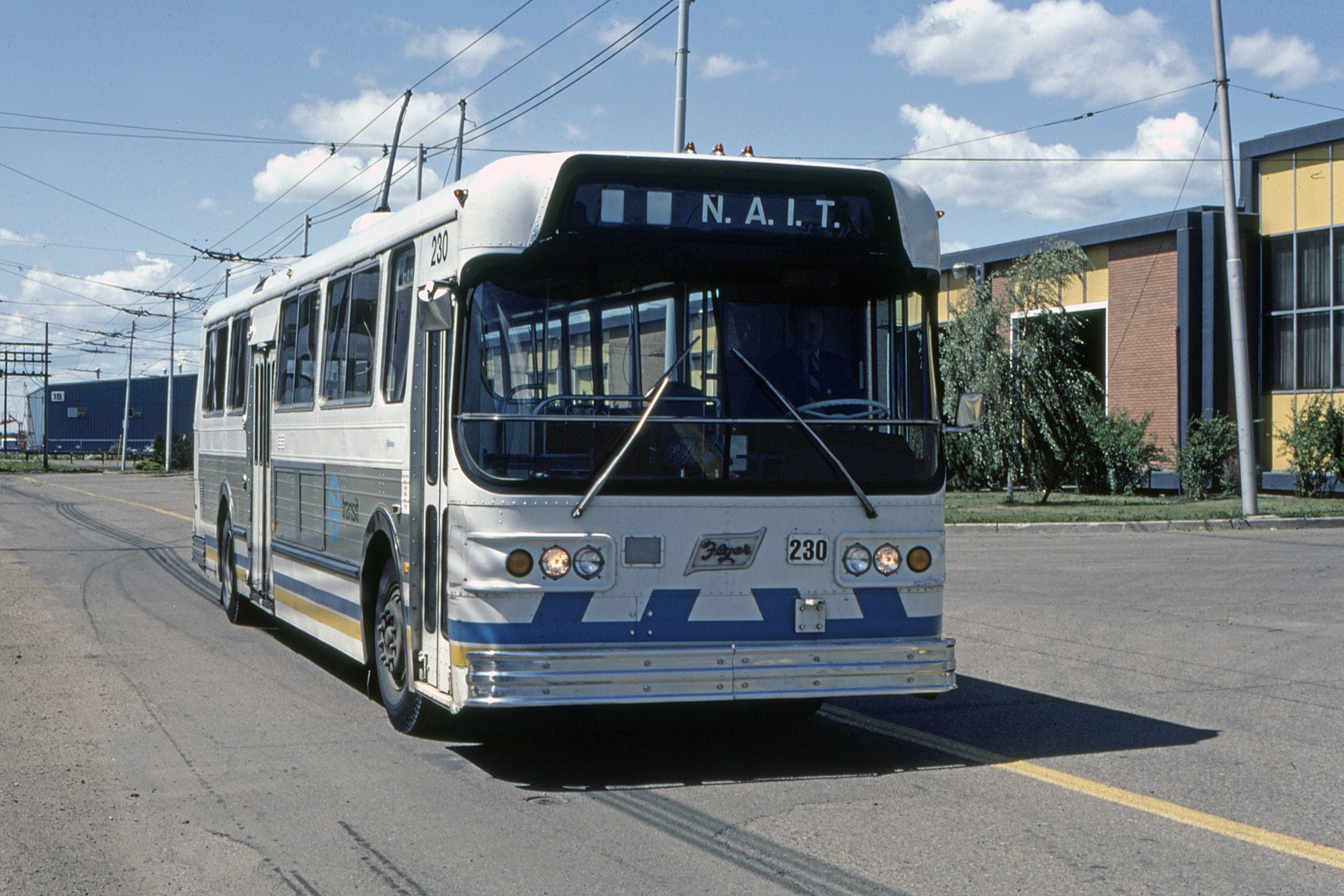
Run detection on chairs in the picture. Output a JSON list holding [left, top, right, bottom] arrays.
[[593, 406, 636, 472], [647, 379, 706, 473], [536, 406, 565, 455], [564, 404, 599, 455]]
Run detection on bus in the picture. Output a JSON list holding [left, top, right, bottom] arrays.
[[191, 146, 981, 737]]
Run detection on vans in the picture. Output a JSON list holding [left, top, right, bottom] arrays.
[[0, 438, 17, 455]]
